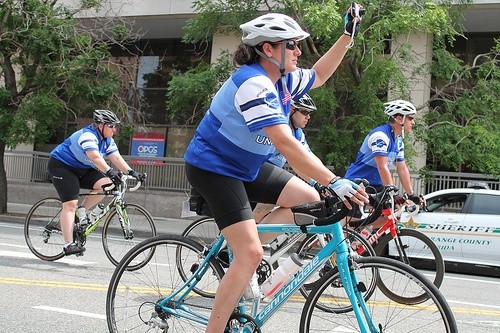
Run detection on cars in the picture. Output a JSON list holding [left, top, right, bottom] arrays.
[[338, 181, 500, 279]]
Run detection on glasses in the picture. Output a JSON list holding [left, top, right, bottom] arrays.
[[404, 117, 414, 121], [104, 124, 116, 128], [259, 41, 301, 50], [294, 109, 313, 117]]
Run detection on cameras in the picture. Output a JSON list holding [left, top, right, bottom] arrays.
[[350, 2, 366, 22]]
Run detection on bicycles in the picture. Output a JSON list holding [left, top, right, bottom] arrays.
[[175, 182, 445, 314], [105, 178, 459, 333], [23, 171, 158, 271]]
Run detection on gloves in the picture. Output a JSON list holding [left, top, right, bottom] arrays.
[[129, 169, 145, 184], [105, 168, 123, 186]]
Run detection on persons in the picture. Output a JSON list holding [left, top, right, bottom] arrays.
[[225, 89, 332, 261], [185, 4, 365, 333], [318, 97, 427, 287], [46, 109, 145, 255]]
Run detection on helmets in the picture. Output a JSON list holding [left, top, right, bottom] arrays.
[[382, 100, 416, 118], [289, 92, 318, 111], [92, 109, 120, 125], [239, 13, 310, 46]]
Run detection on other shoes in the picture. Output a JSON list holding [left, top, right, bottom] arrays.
[[75, 213, 93, 229], [319, 266, 343, 287], [63, 244, 86, 257]]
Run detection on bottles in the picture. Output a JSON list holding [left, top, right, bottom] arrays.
[[78, 204, 88, 225], [244, 270, 259, 300], [350, 224, 373, 251], [260, 251, 306, 299], [89, 203, 104, 220]]
